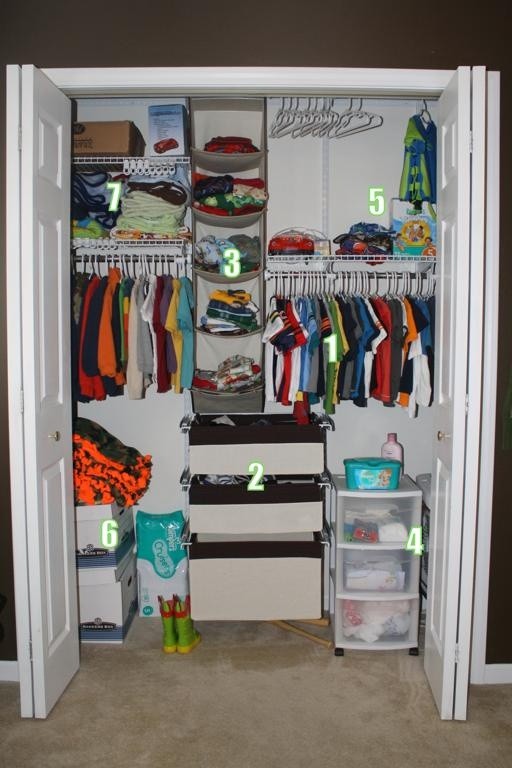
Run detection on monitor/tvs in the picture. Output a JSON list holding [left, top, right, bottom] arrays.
[[389, 197, 438, 261]]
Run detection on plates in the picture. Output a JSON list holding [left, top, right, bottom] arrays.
[[179, 412, 334, 629], [187, 97, 269, 414], [327, 474, 419, 657]]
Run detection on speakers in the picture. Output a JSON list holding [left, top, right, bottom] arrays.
[[174, 601, 202, 655], [159, 600, 175, 654]]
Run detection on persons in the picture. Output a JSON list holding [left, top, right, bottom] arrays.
[[394, 226, 437, 257]]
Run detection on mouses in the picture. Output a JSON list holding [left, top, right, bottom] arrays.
[[268, 96, 383, 139], [271, 269, 435, 301], [419, 97, 433, 123], [71, 248, 192, 282]]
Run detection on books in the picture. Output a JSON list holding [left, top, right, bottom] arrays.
[[71, 120, 146, 157], [77, 551, 137, 644], [416, 474, 434, 600], [148, 103, 188, 156], [342, 458, 401, 491], [76, 502, 137, 568]]
